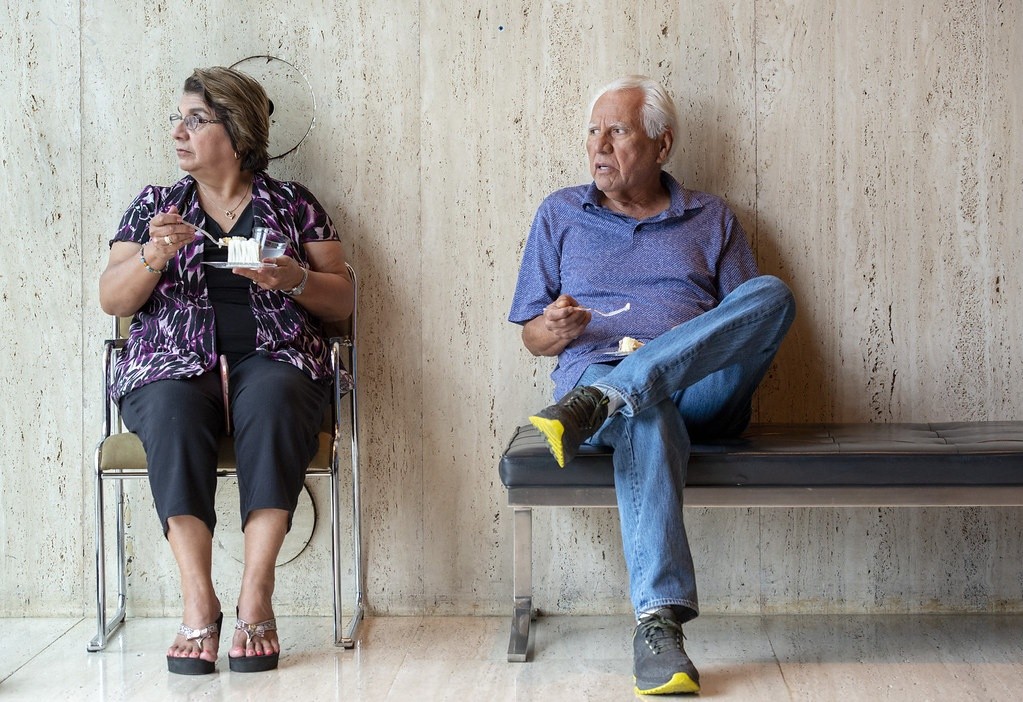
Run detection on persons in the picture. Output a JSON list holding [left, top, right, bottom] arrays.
[[99, 66, 355, 676], [508, 72, 797, 694]]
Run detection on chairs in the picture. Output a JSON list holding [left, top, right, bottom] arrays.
[[86, 260, 364, 656]]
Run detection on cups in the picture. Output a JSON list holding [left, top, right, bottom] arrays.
[[252, 227, 290, 260]]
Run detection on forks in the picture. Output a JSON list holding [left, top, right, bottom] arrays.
[[542, 307, 625, 316]]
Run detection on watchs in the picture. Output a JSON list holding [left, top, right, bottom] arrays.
[[281, 267, 308, 296]]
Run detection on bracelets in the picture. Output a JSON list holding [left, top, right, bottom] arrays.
[[140, 242, 168, 273]]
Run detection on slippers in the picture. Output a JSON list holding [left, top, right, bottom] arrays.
[[167, 611, 223, 675], [228, 605, 279, 672]]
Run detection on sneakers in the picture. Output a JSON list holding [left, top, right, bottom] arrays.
[[631, 607, 700, 694], [528, 385, 611, 468]]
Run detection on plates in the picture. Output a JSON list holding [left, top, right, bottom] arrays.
[[201, 261, 278, 268], [603, 351, 630, 357]]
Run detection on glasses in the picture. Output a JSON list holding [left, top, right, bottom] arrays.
[[169, 114, 224, 130]]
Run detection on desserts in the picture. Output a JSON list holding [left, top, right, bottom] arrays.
[[618, 337, 644, 352], [218, 237, 261, 263]]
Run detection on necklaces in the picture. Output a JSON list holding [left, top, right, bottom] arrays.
[[199, 172, 252, 220]]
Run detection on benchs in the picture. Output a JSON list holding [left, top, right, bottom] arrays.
[[497, 420, 1023, 662]]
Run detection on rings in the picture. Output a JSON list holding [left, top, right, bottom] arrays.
[[164, 236, 172, 245], [253, 281, 257, 284]]
